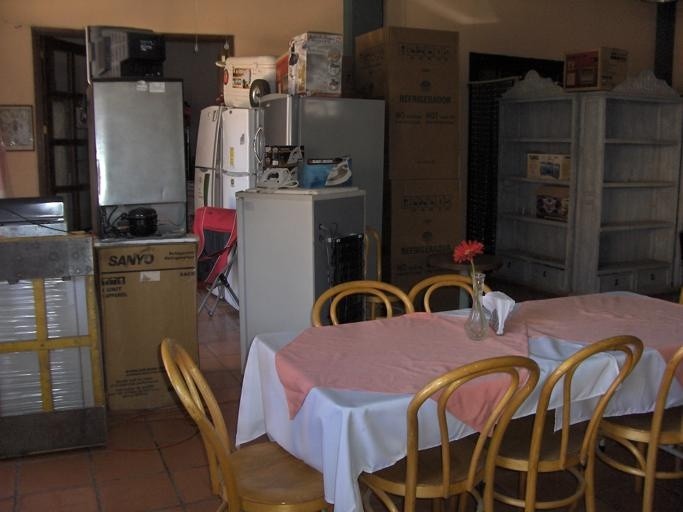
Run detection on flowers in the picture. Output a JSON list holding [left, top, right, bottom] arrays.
[[453, 240, 484, 335]]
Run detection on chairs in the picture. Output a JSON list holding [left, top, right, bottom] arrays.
[[483, 335, 644, 512], [583, 346, 683, 512], [311, 280, 415, 328], [160, 337, 334, 512], [357, 355, 540, 512], [193, 207, 240, 317], [404, 274, 493, 314]]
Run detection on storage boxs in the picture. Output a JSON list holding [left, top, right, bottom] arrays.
[[287, 31, 344, 98], [96, 242, 200, 412], [354, 24, 469, 180], [535, 194, 571, 223], [525, 152, 572, 181], [563, 45, 628, 93], [390, 181, 467, 317]]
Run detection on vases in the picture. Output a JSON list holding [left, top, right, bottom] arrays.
[[463, 271, 489, 341]]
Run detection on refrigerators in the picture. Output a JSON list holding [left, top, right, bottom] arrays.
[[192, 96, 387, 380]]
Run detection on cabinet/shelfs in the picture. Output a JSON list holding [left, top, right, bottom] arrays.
[[571, 69, 683, 298], [494, 69, 581, 296]]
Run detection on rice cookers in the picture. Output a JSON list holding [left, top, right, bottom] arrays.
[[128, 208, 158, 237], [220, 54, 277, 107]]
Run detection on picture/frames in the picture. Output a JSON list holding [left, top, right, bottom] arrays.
[[0, 104, 35, 152]]
